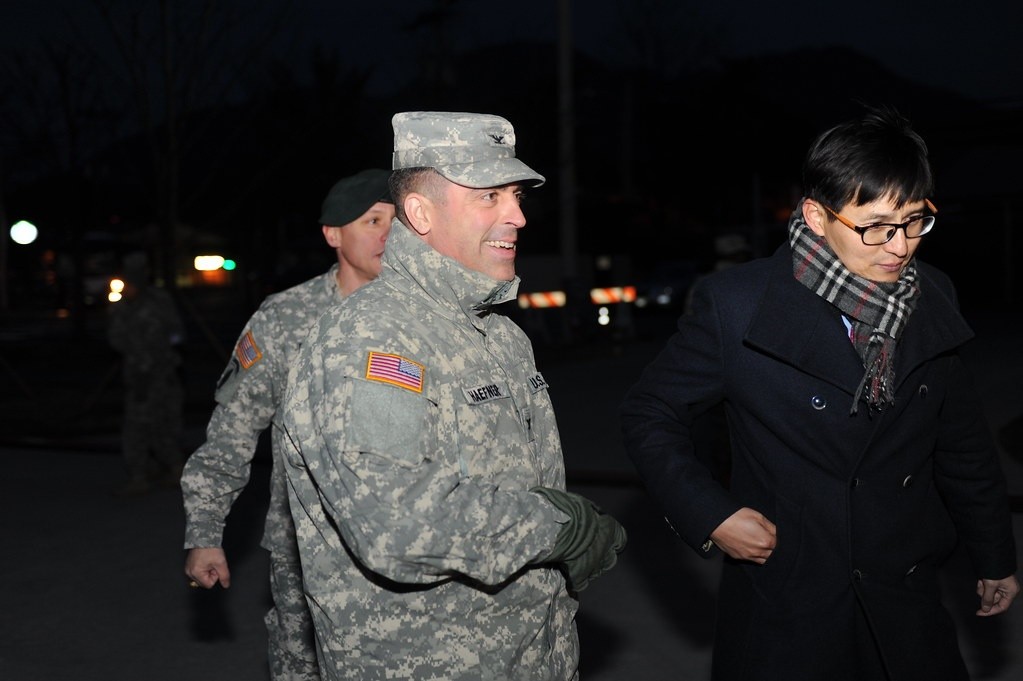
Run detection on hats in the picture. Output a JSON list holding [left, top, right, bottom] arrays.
[[319, 169, 396, 227], [391, 111, 545, 188]]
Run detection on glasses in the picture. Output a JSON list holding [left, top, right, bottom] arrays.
[[824, 198, 940, 246]]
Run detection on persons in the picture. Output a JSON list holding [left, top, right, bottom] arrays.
[[283, 112, 626, 681], [618, 115, 1020, 681], [180, 172, 396, 681], [108, 264, 187, 496]]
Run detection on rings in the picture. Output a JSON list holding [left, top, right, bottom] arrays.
[[190, 581, 197, 587]]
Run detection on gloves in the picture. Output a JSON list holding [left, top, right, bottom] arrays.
[[528, 485, 629, 593]]
